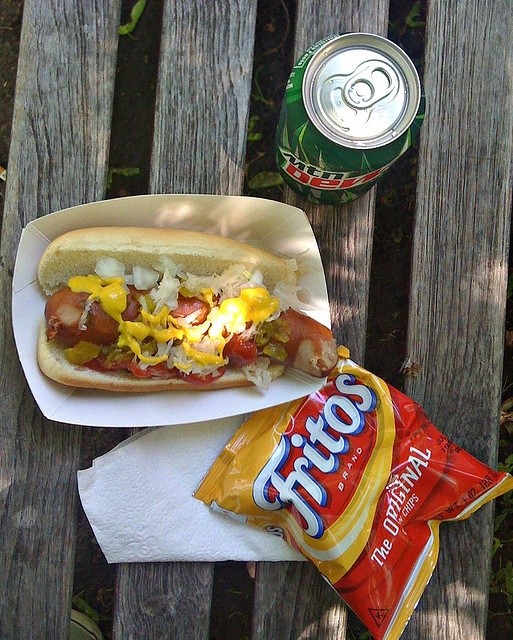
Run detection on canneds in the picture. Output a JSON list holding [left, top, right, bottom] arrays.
[[275, 29, 425, 207]]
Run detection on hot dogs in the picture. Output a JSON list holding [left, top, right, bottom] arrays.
[[37, 223, 339, 396]]
